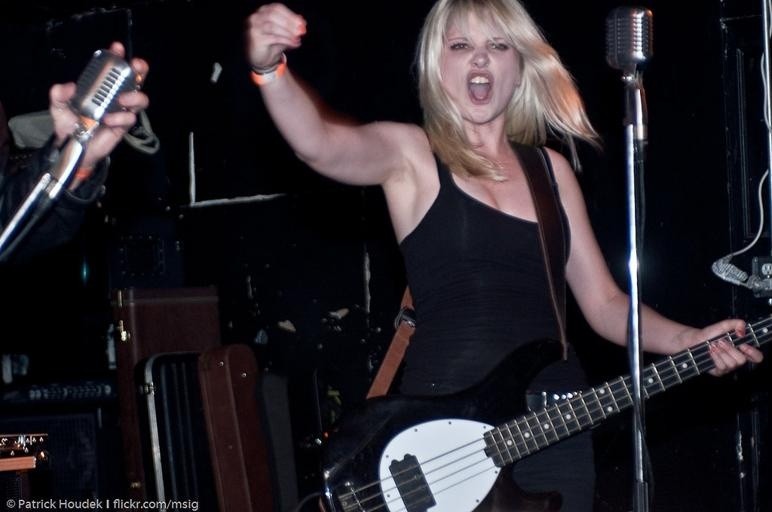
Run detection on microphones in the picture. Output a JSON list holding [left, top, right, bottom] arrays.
[[36, 49, 135, 210], [606, 6, 655, 162]]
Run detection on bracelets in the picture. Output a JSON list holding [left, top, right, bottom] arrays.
[[250, 51, 288, 85]]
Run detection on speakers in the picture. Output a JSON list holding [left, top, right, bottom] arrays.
[[0, 398, 106, 511]]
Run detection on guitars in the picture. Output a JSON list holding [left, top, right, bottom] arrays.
[[291, 316, 770, 510]]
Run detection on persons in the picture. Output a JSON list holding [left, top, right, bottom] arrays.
[[247, 0, 765, 512], [1, 42, 150, 260]]
